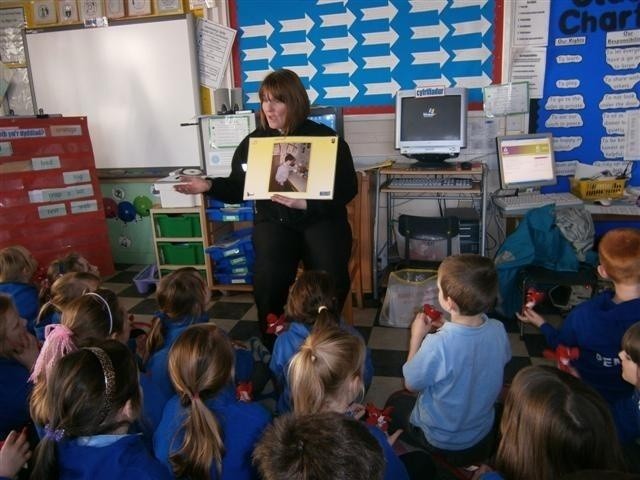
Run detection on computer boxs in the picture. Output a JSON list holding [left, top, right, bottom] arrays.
[[444, 207, 480, 255]]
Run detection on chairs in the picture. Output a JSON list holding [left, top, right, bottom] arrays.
[[296, 172, 363, 327], [373, 214, 460, 327]]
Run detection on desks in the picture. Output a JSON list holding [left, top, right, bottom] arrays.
[[493, 193, 639, 245], [373, 162, 489, 301]]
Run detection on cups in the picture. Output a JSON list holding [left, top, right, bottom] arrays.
[[231, 87, 243, 114], [214, 89, 230, 114]]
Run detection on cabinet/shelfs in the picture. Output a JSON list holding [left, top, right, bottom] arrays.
[[201, 166, 374, 308], [148, 207, 211, 294]]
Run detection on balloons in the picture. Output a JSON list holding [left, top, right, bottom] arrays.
[[102, 197, 118, 222], [117, 201, 137, 225], [133, 195, 153, 219]]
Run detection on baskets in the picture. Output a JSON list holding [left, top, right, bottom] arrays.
[[569, 175, 627, 202]]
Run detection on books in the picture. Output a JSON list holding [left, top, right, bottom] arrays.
[[243, 136, 339, 201]]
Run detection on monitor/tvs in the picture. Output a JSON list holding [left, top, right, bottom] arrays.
[[496, 132, 558, 196], [394, 87, 468, 167], [306, 106, 345, 143]]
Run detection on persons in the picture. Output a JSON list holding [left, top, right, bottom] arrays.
[[383, 252, 512, 456], [273, 155, 303, 183], [515, 227, 640, 399], [0, 245, 437, 479], [173, 68, 358, 353], [473, 363, 630, 480], [607, 321, 640, 444]]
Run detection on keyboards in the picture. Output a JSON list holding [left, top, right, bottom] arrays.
[[386, 178, 473, 189], [492, 191, 584, 211]]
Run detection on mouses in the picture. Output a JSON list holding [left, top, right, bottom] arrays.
[[461, 161, 472, 170], [593, 199, 611, 207]]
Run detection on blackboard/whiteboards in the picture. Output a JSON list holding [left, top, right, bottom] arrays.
[[21, 13, 205, 179]]
